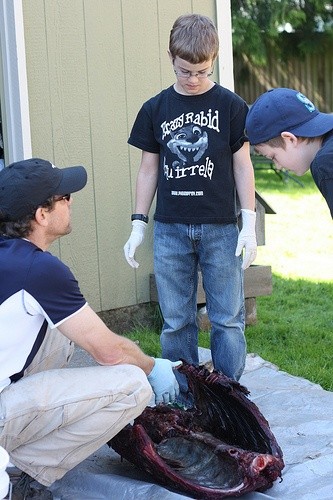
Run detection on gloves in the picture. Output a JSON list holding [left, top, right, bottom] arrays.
[[123, 220, 148, 269], [235, 209, 257, 270], [147, 357, 183, 405]]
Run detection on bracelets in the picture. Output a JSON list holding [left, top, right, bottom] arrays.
[[131, 214, 148, 223]]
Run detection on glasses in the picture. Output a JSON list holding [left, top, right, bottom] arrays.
[[172, 59, 215, 78], [54, 193, 71, 202]]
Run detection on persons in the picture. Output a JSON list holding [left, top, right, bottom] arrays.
[[244, 87, 333, 219], [123, 14, 259, 382], [0, 158, 183, 500]]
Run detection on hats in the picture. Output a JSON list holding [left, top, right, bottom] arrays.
[[0, 158, 88, 221], [245, 88, 333, 145]]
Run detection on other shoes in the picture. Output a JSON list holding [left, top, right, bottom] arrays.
[[5, 466, 53, 500]]
